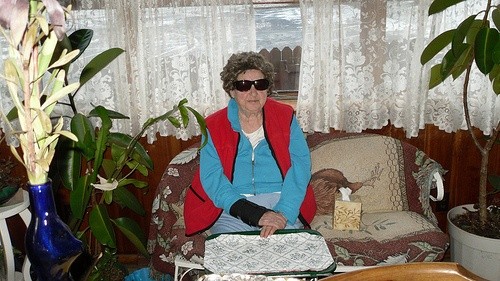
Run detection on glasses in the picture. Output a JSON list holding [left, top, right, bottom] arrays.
[[235, 78, 269, 91]]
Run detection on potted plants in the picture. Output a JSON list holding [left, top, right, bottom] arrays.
[[419, 0, 500, 281]]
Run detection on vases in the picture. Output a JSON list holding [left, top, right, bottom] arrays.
[[27, 178, 83, 268]]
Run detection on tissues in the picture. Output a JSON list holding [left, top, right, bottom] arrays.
[[333, 187, 361, 230]]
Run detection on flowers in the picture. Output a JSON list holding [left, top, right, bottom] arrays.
[[0, 0, 81, 185], [91, 174, 118, 191]]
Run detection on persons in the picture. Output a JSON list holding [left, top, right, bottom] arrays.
[[183, 51, 318, 239]]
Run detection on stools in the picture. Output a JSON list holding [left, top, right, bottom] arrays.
[[0, 187, 39, 281]]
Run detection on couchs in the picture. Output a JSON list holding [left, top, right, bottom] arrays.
[[147, 129, 449, 281]]
